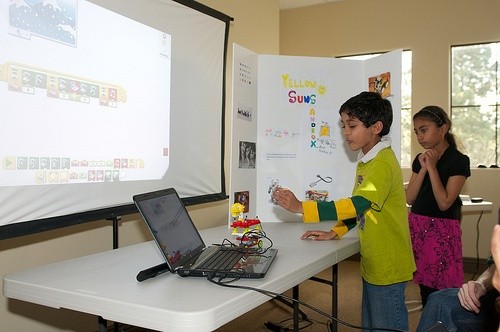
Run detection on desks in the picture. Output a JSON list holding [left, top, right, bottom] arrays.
[[4, 219, 360, 332], [407, 201, 493, 213]]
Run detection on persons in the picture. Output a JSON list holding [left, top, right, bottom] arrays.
[[405, 105, 472, 312], [415, 205, 500, 332], [272, 91, 417, 332], [457, 264, 496, 314]]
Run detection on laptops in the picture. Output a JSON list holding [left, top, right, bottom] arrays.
[[133, 188, 278, 278]]
